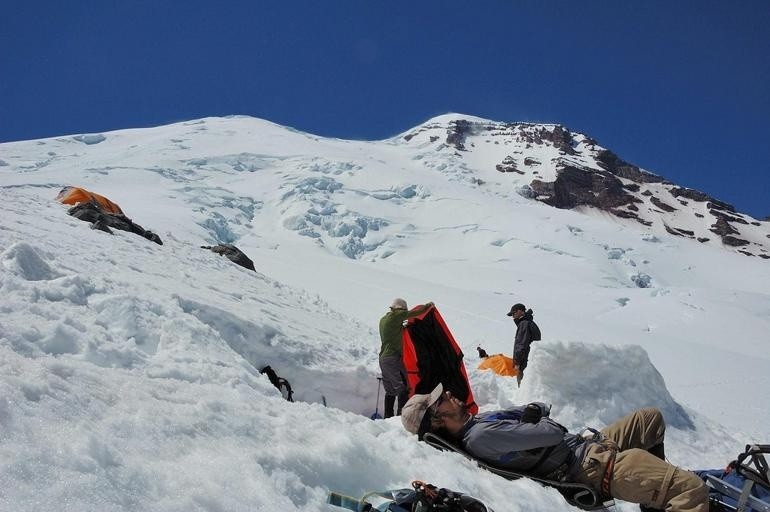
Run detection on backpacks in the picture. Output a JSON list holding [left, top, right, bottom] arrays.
[[694, 444, 769, 512], [325, 481, 487, 512], [261, 366, 294, 403], [528, 321, 541, 341]]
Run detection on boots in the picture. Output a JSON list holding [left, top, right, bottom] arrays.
[[384, 392, 410, 419]]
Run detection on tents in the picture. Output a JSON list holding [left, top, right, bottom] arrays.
[[55, 185, 124, 215], [478, 352, 519, 377], [689, 467, 770, 512]]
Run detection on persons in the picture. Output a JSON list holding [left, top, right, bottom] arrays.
[[401, 382, 709, 512], [379, 298, 433, 419], [506, 304, 541, 384], [478, 347, 488, 358]]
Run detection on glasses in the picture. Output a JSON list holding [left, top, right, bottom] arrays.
[[437, 397, 443, 407]]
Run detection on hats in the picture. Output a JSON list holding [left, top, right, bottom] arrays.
[[389, 298, 407, 310], [506, 303, 526, 316], [401, 382, 443, 434]]
